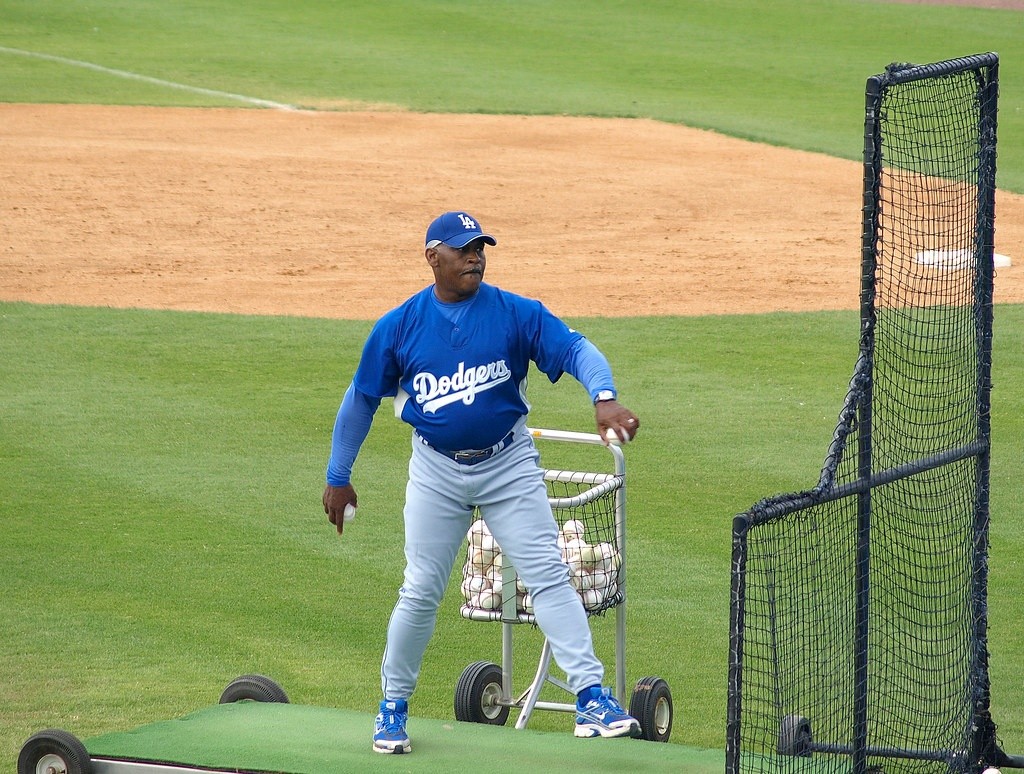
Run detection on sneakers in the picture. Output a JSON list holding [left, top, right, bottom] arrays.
[[573, 689, 642, 738], [372, 700, 412, 754]]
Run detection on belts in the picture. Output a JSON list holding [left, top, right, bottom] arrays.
[[431, 430, 514, 466]]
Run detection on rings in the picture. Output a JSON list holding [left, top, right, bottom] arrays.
[[627, 417, 635, 423]]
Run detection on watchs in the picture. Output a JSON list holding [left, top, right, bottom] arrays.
[[594, 390, 616, 406]]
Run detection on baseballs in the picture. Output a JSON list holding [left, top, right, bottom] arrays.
[[461, 518, 622, 614], [343, 503, 356, 521]]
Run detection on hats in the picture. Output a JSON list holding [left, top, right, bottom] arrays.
[[425, 211, 497, 249]]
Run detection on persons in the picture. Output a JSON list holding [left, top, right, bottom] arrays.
[[321, 211, 643, 754]]
